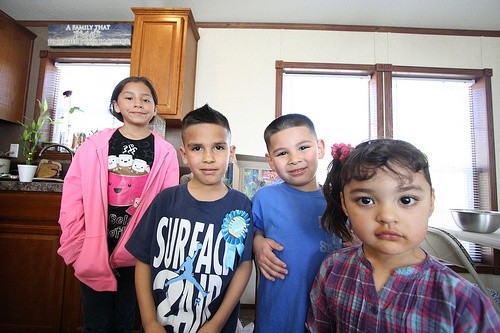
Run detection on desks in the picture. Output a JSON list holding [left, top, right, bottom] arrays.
[[440, 230, 500, 252]]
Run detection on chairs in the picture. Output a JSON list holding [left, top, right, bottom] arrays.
[[419, 224, 500, 319]]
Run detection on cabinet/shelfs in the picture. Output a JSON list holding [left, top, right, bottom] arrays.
[[0, 180, 81, 333], [0, 11, 39, 125], [130, 7, 201, 124]]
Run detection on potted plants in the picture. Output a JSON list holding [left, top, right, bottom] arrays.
[[0, 150, 15, 174], [17, 97, 62, 182]]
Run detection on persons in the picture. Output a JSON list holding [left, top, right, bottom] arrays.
[[303, 139, 500, 333], [249, 114, 362, 333], [124, 103, 254, 333], [58, 76, 180, 332]]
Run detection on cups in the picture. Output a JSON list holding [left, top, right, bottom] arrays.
[[18, 164, 38, 182], [0, 159, 12, 174]]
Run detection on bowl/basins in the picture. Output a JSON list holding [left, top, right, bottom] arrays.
[[450, 209, 500, 234]]
